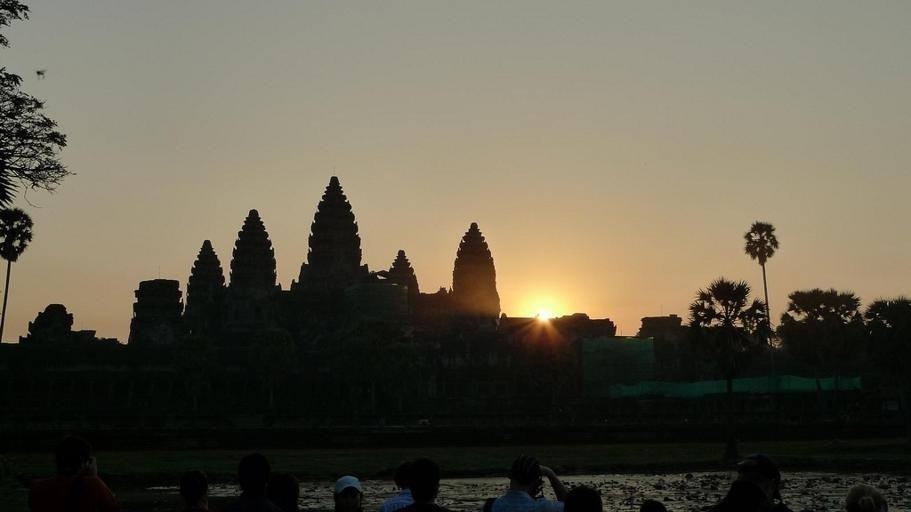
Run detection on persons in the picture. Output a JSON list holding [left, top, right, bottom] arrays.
[[238, 454, 281, 510], [28, 450, 116, 509], [378, 461, 417, 510], [636, 498, 669, 511], [560, 484, 605, 511], [694, 453, 793, 511], [401, 457, 448, 510], [843, 480, 888, 512], [474, 456, 571, 511], [266, 473, 300, 510], [333, 476, 365, 510], [179, 470, 209, 510]]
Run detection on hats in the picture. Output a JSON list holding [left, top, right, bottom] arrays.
[[334, 475, 363, 495], [740, 454, 783, 502]]
